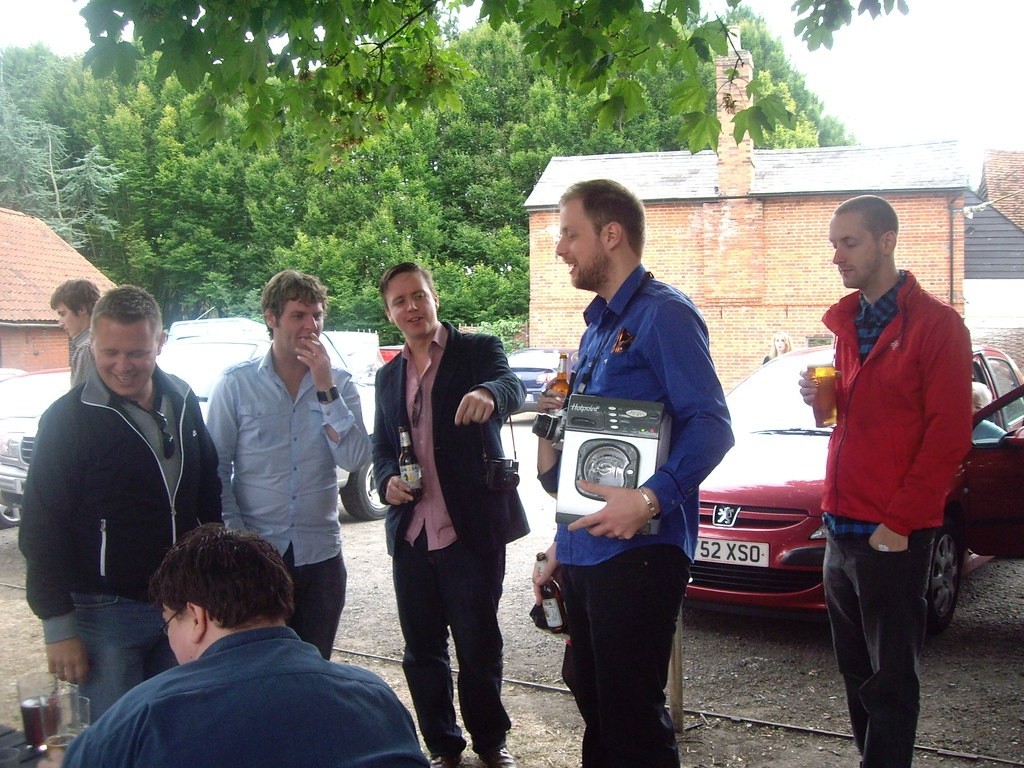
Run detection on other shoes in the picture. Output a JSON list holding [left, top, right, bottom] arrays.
[[430, 753, 463, 768], [479, 747, 516, 768]]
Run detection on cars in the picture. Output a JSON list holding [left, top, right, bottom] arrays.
[[685, 343, 1024, 641], [503, 348, 580, 424], [0, 368, 27, 528], [0, 332, 390, 527]]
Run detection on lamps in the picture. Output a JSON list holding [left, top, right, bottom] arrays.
[[965, 226, 975, 236]]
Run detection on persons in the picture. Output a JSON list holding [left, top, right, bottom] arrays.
[[372, 262, 530, 768], [798, 195, 973, 768], [762, 330, 793, 366], [207, 270, 373, 660], [60, 523, 432, 768], [19, 285, 225, 727], [972, 382, 1008, 440], [51, 279, 102, 390], [532, 179, 735, 768]]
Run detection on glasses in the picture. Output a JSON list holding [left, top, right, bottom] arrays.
[[160, 607, 185, 636], [151, 409, 175, 459], [410, 386, 424, 429]]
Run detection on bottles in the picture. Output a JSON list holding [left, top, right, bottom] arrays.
[[536, 553, 567, 633], [546, 352, 569, 414], [397, 425, 424, 499]]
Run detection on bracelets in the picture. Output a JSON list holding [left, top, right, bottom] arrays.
[[316, 385, 339, 403], [638, 487, 656, 516]]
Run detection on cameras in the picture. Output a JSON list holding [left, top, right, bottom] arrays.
[[485, 457, 520, 492], [532, 413, 565, 451]]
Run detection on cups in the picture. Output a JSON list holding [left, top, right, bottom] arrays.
[[807, 363, 838, 428], [16, 670, 59, 748], [0, 747, 22, 767], [56, 696, 91, 752], [38, 681, 79, 757]]
